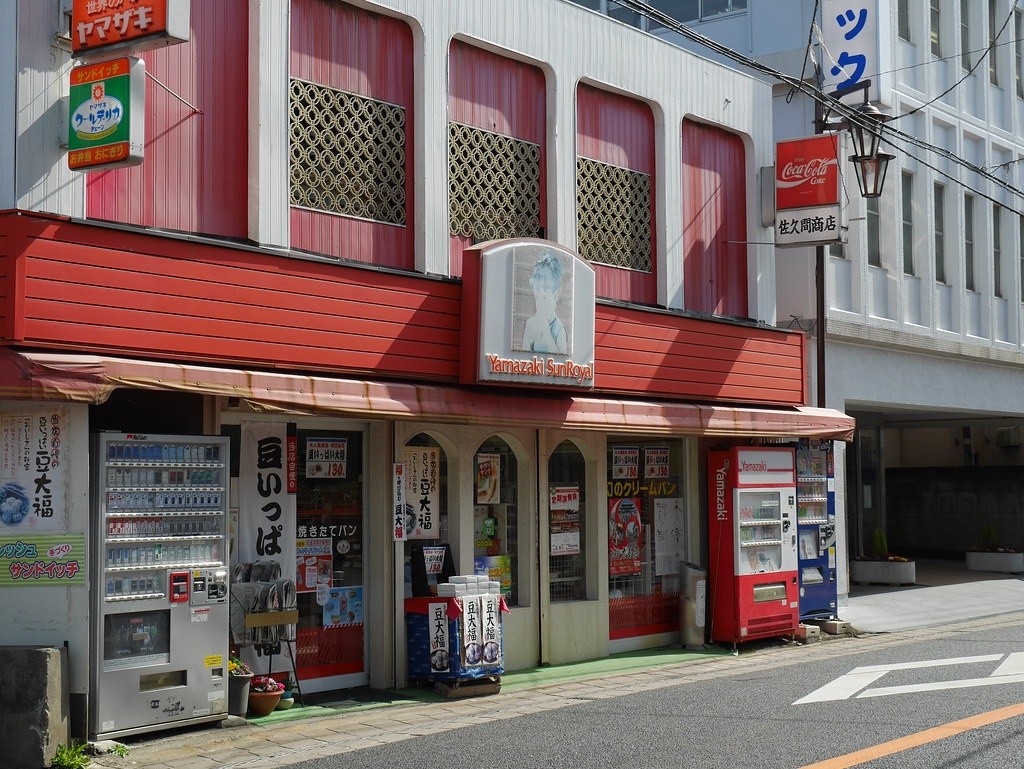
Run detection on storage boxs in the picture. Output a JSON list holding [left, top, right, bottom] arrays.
[[489, 581, 500, 588], [465, 575, 489, 582], [490, 588, 500, 594], [467, 589, 479, 595], [437, 582, 466, 592], [438, 590, 467, 597], [477, 582, 489, 589], [449, 576, 477, 584], [478, 588, 490, 594], [466, 583, 477, 590]]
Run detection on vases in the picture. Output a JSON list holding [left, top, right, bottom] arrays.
[[965, 552, 1024, 575], [248, 689, 285, 717], [228, 670, 254, 718], [851, 560, 916, 586], [277, 697, 294, 710]]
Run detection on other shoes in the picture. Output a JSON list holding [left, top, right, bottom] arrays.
[[967, 543, 1022, 553]]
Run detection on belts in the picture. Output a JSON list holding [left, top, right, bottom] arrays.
[[234, 560, 295, 657]]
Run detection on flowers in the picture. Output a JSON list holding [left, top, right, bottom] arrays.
[[852, 526, 912, 563], [228, 650, 250, 675], [968, 520, 1022, 553], [249, 676, 285, 693]]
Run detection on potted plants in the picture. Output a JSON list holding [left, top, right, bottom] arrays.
[[277, 676, 299, 698]]
